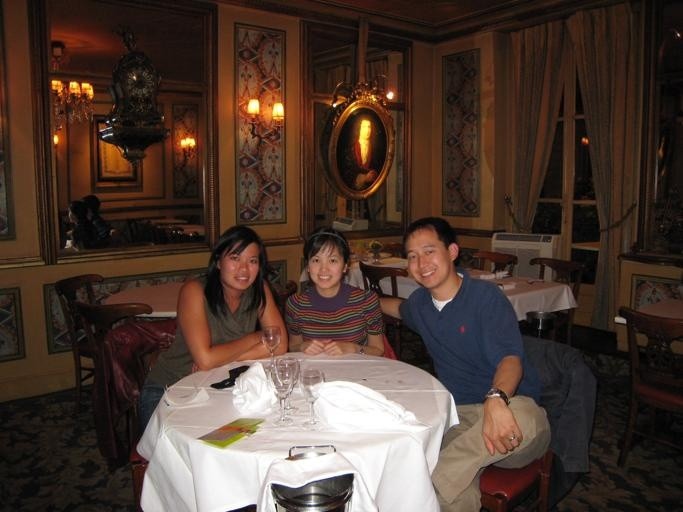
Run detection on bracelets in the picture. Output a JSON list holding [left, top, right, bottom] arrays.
[[359, 344, 366, 357]]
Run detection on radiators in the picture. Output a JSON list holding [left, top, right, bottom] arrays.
[[331, 218, 368, 231], [490, 234, 564, 284]]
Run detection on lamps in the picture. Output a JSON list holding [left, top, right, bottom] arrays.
[[247, 99, 285, 137], [50, 41, 94, 127], [180, 136, 197, 160]]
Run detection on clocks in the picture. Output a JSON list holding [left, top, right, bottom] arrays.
[[95, 27, 169, 166]]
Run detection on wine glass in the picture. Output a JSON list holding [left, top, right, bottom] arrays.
[[269, 357, 325, 431], [261, 325, 281, 372]]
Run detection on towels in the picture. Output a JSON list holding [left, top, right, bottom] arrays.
[[311, 381, 416, 427], [251, 453, 380, 512], [232, 362, 277, 418]]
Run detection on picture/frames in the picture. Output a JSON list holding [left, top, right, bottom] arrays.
[[328, 91, 394, 201]]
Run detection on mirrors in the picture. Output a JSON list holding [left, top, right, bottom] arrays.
[[306, 32, 406, 230], [28, 0, 220, 267]]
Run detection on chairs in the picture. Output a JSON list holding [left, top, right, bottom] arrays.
[[127, 217, 200, 244], [617, 309, 682, 476], [358, 259, 408, 355], [473, 336, 597, 512], [111, 322, 181, 507], [55, 275, 153, 414], [528, 257, 585, 334], [468, 251, 517, 278]]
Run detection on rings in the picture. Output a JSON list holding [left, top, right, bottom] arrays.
[[507, 435, 516, 443]]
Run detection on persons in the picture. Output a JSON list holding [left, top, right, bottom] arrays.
[[81, 195, 113, 239], [283, 227, 384, 357], [341, 117, 377, 189], [66, 199, 97, 236], [377, 217, 551, 511], [135, 225, 287, 431]]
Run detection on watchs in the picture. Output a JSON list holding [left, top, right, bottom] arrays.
[[482, 388, 509, 406]]
